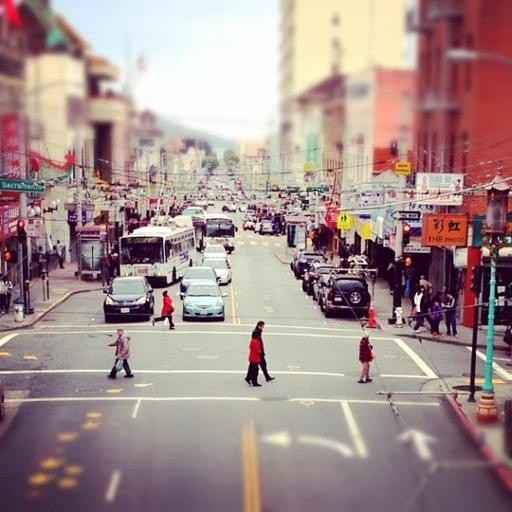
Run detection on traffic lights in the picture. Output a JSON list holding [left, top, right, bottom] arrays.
[[4, 238, 16, 262], [404, 255, 412, 268], [402, 224, 411, 245], [18, 220, 26, 238]]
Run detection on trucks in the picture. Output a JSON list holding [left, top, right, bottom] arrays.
[[180, 207, 207, 228]]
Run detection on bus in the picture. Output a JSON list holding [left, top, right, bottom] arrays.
[[112, 214, 200, 289], [202, 213, 238, 255]]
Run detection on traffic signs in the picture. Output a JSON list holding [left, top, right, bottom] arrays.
[[0, 179, 46, 194], [389, 210, 420, 221]]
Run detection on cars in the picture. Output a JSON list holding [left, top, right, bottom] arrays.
[[201, 258, 233, 285], [253, 222, 261, 234], [177, 265, 221, 300], [100, 275, 155, 324], [0, 376, 6, 420], [202, 244, 230, 264], [205, 165, 254, 232], [179, 282, 227, 321], [290, 249, 370, 317]]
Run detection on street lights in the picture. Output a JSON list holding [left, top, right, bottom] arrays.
[[477, 162, 512, 424], [29, 198, 62, 299]]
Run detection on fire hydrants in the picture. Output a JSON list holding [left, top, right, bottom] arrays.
[[393, 306, 403, 328]]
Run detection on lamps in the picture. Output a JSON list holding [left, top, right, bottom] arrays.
[[28, 200, 60, 216]]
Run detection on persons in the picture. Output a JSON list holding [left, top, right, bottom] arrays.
[[108, 330, 133, 380], [388, 265, 457, 336], [56, 240, 64, 268], [3, 276, 13, 313], [358, 336, 374, 384], [100, 249, 119, 293], [151, 291, 175, 330], [38, 245, 47, 278], [253, 321, 275, 382], [245, 331, 262, 386], [0, 273, 5, 314]]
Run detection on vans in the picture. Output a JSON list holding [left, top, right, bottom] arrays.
[[195, 199, 208, 211], [260, 219, 274, 235]]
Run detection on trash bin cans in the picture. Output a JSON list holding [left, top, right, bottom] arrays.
[[12, 298, 23, 323]]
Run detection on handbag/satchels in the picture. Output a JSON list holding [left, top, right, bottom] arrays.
[[116, 358, 124, 371]]
